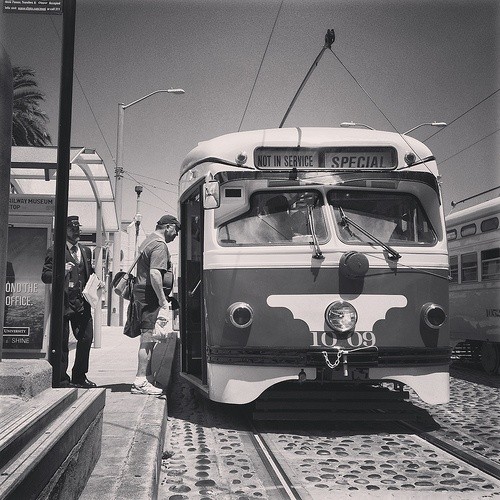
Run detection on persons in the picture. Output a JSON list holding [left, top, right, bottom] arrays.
[[41, 216, 106, 387], [130, 215, 181, 393], [361, 207, 405, 242], [289, 210, 319, 239], [258, 197, 292, 245], [4, 261, 16, 325], [310, 206, 327, 241]]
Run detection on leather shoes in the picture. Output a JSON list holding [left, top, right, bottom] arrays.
[[74, 377, 97, 388], [63, 381, 78, 388]]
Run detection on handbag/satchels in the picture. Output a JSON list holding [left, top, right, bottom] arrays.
[[123, 301, 141, 338], [112, 272, 136, 300], [153, 302, 174, 341]]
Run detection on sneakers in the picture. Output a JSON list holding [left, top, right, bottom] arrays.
[[131, 379, 163, 395], [151, 379, 167, 390]]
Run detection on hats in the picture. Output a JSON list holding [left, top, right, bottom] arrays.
[[66, 216, 82, 226], [158, 215, 182, 230]]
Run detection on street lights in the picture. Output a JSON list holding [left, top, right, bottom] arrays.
[[110, 88, 185, 327], [133, 211, 143, 277]]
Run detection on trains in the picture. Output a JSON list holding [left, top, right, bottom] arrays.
[[441, 193, 500, 372], [175, 127, 451, 406]]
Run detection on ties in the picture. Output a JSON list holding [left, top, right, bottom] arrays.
[[72, 247, 79, 263]]
